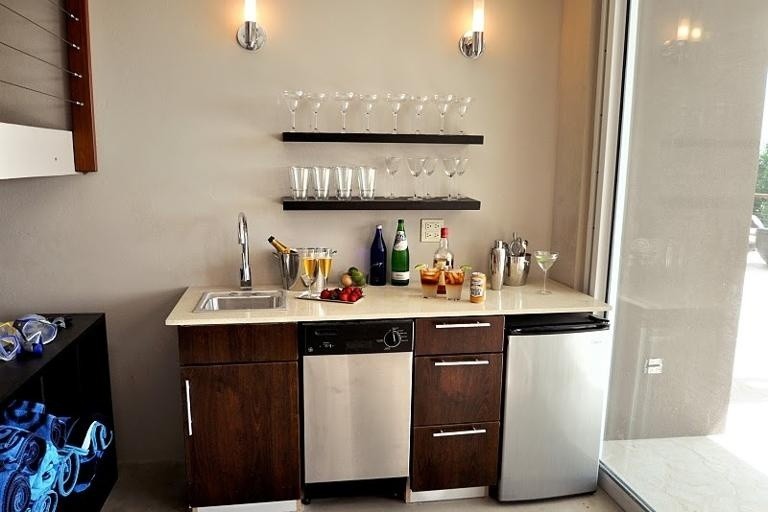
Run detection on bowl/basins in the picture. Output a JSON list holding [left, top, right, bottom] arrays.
[[338, 272, 368, 288]]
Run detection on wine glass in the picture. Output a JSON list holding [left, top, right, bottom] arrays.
[[383, 152, 470, 203], [314, 247, 333, 299], [534, 250, 560, 297], [300, 248, 318, 298], [282, 87, 475, 136]]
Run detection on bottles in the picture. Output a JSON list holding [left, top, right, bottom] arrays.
[[267, 236, 298, 253], [433, 227, 455, 295], [368, 224, 388, 286], [489, 240, 508, 292], [390, 218, 412, 288]]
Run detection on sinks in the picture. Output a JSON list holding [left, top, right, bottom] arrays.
[[189, 285, 288, 314]]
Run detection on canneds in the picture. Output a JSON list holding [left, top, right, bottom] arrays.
[[470, 272, 486, 303]]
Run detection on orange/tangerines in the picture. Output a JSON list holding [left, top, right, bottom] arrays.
[[348, 267, 365, 285]]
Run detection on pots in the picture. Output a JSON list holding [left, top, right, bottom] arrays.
[[268, 247, 337, 293]]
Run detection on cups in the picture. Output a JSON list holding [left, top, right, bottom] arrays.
[[418, 263, 442, 298], [284, 164, 375, 201], [442, 265, 466, 301], [504, 253, 532, 287]]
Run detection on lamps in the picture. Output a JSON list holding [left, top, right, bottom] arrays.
[[458, 0, 487, 59], [235, 0, 266, 51], [662, 14, 702, 66]]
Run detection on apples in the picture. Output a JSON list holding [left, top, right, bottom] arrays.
[[341, 274, 353, 286]]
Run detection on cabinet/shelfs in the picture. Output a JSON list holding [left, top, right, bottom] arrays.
[[180, 324, 301, 507], [411, 318, 505, 490], [277, 131, 484, 211], [0, 311, 119, 512]]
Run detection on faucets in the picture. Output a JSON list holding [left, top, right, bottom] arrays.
[[234, 210, 251, 288]]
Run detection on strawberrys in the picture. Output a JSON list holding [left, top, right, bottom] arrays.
[[320, 287, 364, 302]]
